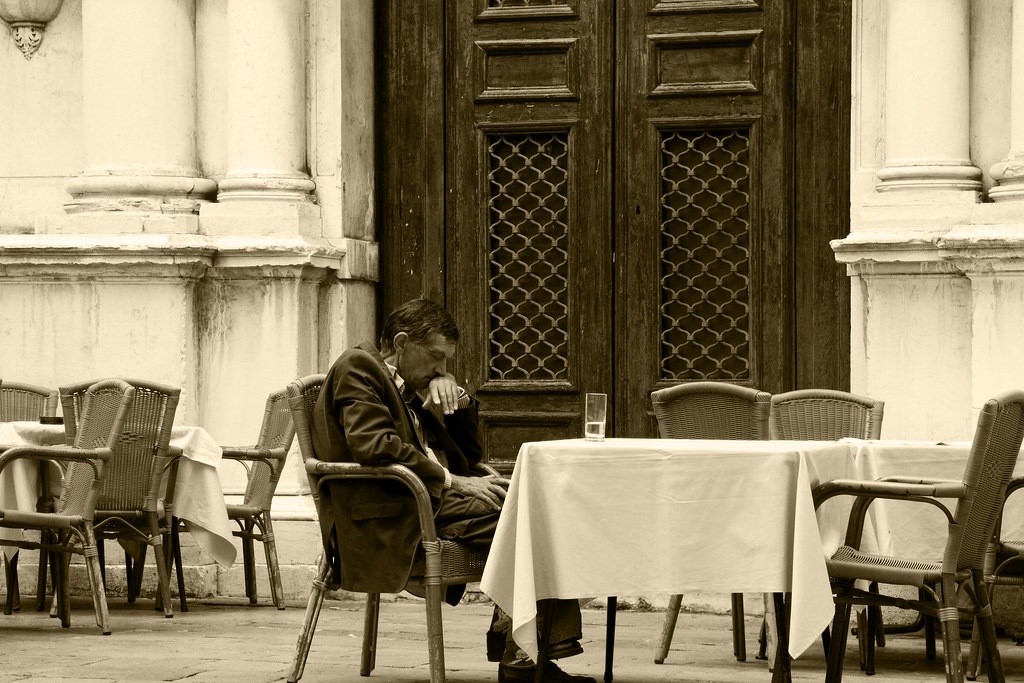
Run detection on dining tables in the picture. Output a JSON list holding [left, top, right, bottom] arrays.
[[478, 434, 1024, 683]]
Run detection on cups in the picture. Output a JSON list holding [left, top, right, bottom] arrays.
[[584, 393, 607, 443]]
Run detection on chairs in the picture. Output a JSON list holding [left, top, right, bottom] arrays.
[[1, 376, 300, 634], [650, 381, 772, 664], [966, 476, 1024, 680], [771, 388, 1024, 682], [287, 372, 495, 683], [755, 389, 888, 664]]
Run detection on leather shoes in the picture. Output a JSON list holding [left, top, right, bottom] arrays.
[[497, 662, 597, 683], [486, 630, 584, 663]]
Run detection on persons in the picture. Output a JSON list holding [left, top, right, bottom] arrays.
[[310, 296, 599, 683]]
